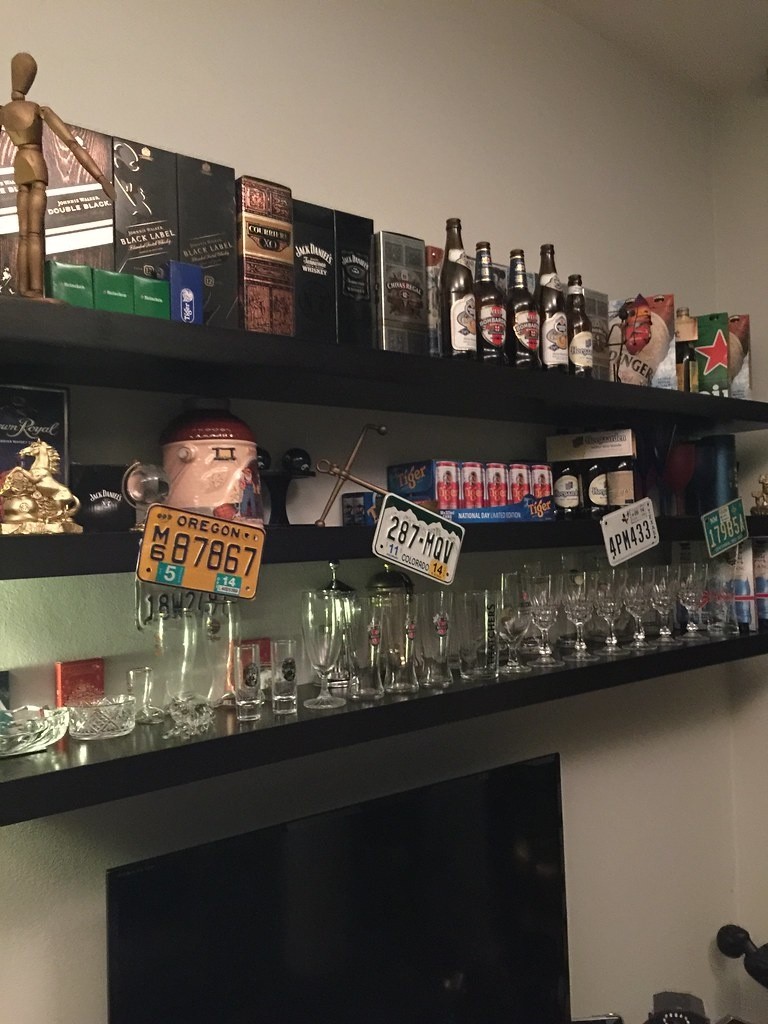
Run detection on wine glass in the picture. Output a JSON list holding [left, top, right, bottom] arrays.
[[622, 566, 659, 651], [593, 567, 631, 657], [650, 564, 682, 646], [561, 572, 600, 663], [525, 572, 565, 668], [676, 563, 710, 642], [303, 590, 348, 709], [198, 600, 239, 710], [159, 608, 200, 739], [496, 572, 533, 674]]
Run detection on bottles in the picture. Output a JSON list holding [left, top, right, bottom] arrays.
[[537, 245, 568, 372], [608, 414, 640, 512], [566, 274, 594, 377], [553, 422, 582, 520], [580, 419, 609, 518], [507, 249, 540, 367], [439, 218, 477, 358], [673, 308, 699, 393], [475, 242, 509, 364]]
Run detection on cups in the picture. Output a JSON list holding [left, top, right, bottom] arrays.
[[234, 643, 261, 721], [129, 666, 153, 724], [344, 593, 384, 703], [416, 590, 453, 690], [380, 592, 419, 695], [707, 561, 738, 636], [456, 589, 500, 681], [271, 640, 297, 715]]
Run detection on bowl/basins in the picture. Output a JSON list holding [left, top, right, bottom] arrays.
[[0, 706, 70, 757], [66, 694, 136, 741]]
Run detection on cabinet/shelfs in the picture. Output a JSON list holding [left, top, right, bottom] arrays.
[[0, 293, 768, 827]]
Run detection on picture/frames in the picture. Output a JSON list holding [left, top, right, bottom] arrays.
[[0, 384, 71, 488]]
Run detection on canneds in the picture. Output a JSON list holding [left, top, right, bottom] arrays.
[[462, 462, 484, 509], [436, 460, 459, 509], [531, 465, 551, 499], [486, 463, 508, 507], [510, 464, 530, 504]]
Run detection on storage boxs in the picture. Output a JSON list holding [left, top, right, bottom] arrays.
[[387, 461, 558, 525], [0, 105, 611, 379]]
